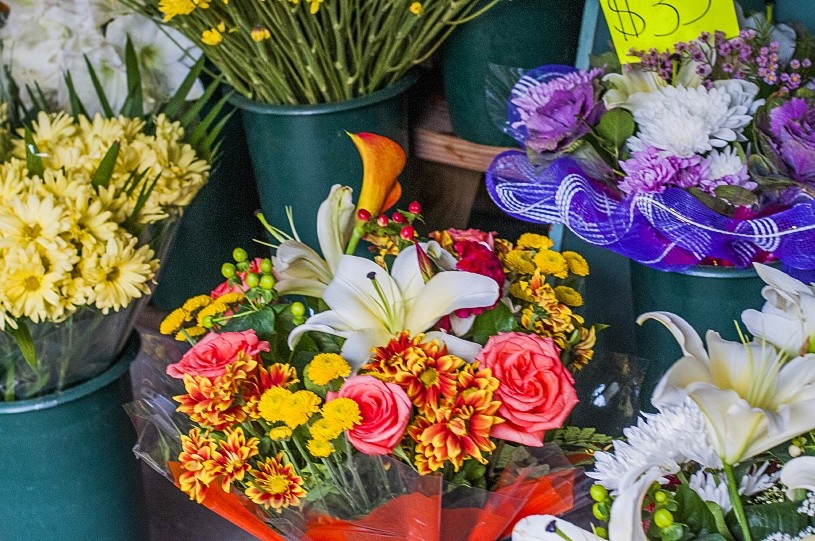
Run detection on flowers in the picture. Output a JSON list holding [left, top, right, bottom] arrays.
[[1, 0, 815, 540]]
